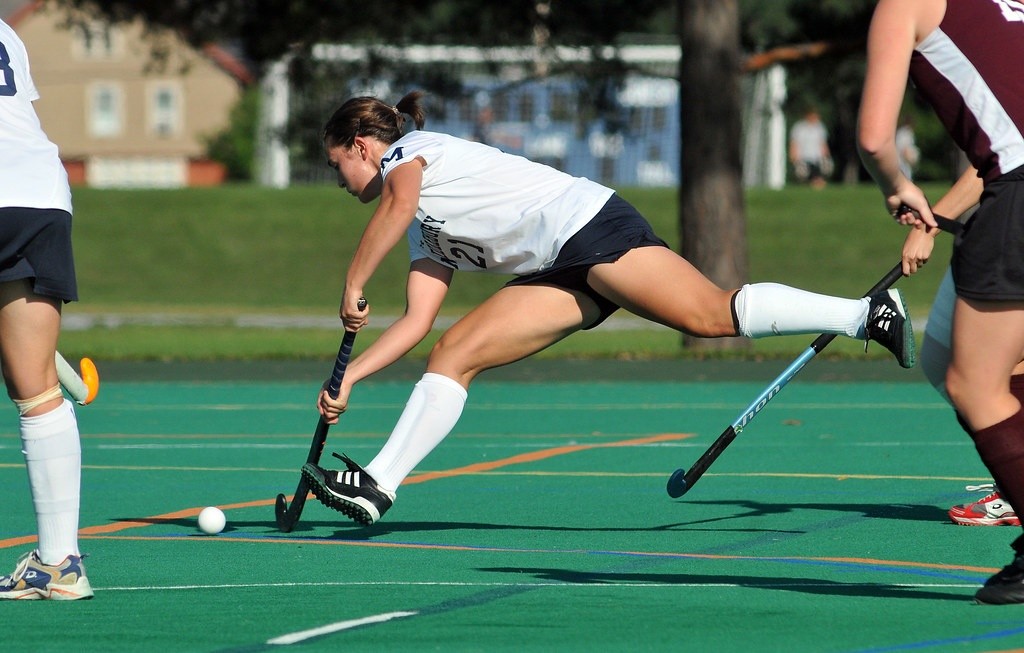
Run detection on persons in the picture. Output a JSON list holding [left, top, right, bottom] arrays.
[[895, 121, 920, 179], [857, 0, 1024, 605], [0, 21, 93, 601], [302, 92, 915, 525], [788, 111, 834, 188]]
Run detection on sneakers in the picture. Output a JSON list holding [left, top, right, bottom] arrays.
[[865, 288, 916, 369], [948, 483, 1023, 526], [0, 550, 94, 600], [975, 533, 1024, 605], [300, 452, 396, 528]]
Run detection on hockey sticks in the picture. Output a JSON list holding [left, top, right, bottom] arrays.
[[665, 258, 910, 500], [273, 295, 368, 534], [54, 349, 100, 406], [897, 202, 964, 236]]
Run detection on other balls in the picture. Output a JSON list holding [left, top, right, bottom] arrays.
[[198, 506, 227, 534]]
